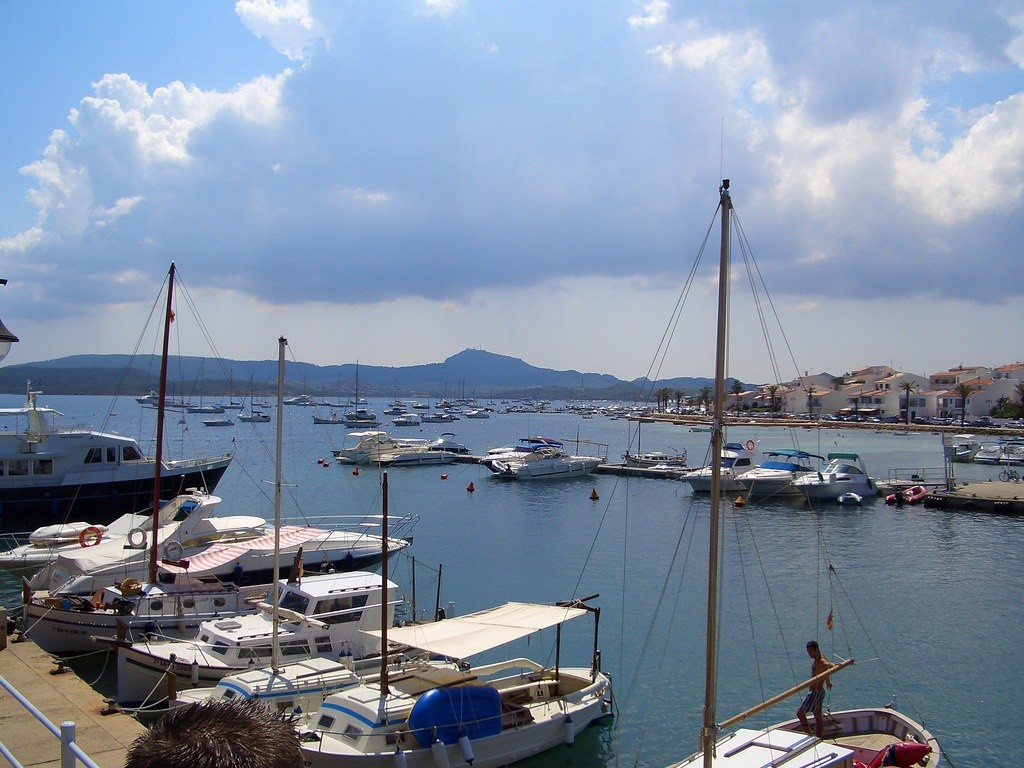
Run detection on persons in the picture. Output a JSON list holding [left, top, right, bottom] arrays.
[[896, 488, 903, 509], [234, 562, 243, 585], [346, 551, 353, 571], [797, 641, 832, 742], [124, 693, 315, 768]]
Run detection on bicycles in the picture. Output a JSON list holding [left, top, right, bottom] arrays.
[[998, 464, 1020, 483]]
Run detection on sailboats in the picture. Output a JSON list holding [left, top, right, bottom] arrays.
[[237, 372, 271, 423], [512, 173, 948, 768], [338, 358, 382, 429], [136, 357, 227, 414], [167, 337, 464, 736]]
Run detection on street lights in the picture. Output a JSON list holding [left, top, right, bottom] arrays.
[[221, 368, 246, 410]]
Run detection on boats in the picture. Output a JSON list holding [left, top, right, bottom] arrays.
[[479, 432, 603, 482], [973, 440, 1006, 465], [90, 570, 412, 712], [883, 485, 927, 506], [262, 405, 273, 409], [202, 420, 235, 426], [1000, 441, 1024, 464], [464, 376, 654, 422], [731, 449, 825, 495], [0, 376, 236, 532], [947, 439, 981, 462], [789, 452, 879, 500], [620, 449, 706, 478], [680, 443, 760, 492], [1, 259, 474, 655], [313, 413, 343, 424], [251, 401, 266, 406], [343, 409, 377, 421], [392, 414, 421, 426], [287, 466, 613, 768]]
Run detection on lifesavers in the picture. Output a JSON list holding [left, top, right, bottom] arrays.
[[127, 527, 147, 548], [78, 527, 101, 548], [746, 440, 756, 451], [164, 541, 183, 563]]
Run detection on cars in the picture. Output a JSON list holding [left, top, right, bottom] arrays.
[[912, 416, 1024, 429], [668, 407, 906, 424]]
